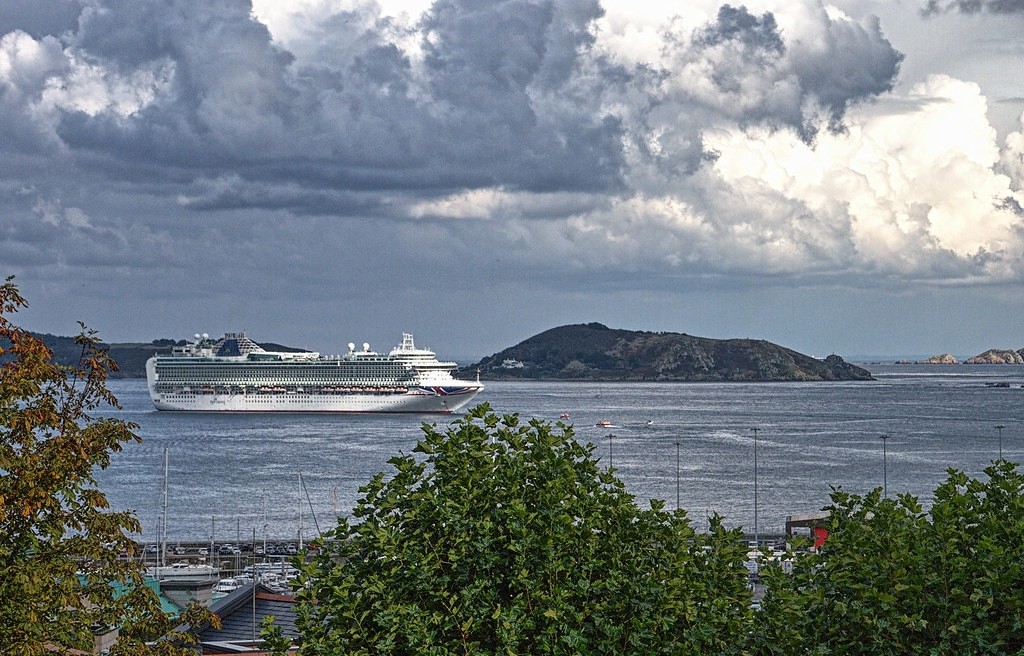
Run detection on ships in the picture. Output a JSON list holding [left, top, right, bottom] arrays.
[[145, 329, 485, 414]]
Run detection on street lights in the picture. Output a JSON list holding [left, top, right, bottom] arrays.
[[673, 441, 683, 515], [879, 433, 890, 499], [748, 426, 762, 547], [993, 424, 1007, 475], [603, 434, 618, 492]]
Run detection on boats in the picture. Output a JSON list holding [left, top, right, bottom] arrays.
[[379, 387, 408, 393], [260, 387, 272, 392], [350, 387, 363, 393], [273, 386, 285, 392], [335, 387, 350, 394], [297, 387, 303, 391], [136, 446, 369, 599], [559, 412, 569, 420], [365, 387, 378, 392], [201, 386, 213, 392], [287, 390, 295, 394], [596, 420, 615, 429], [321, 387, 334, 393]]
